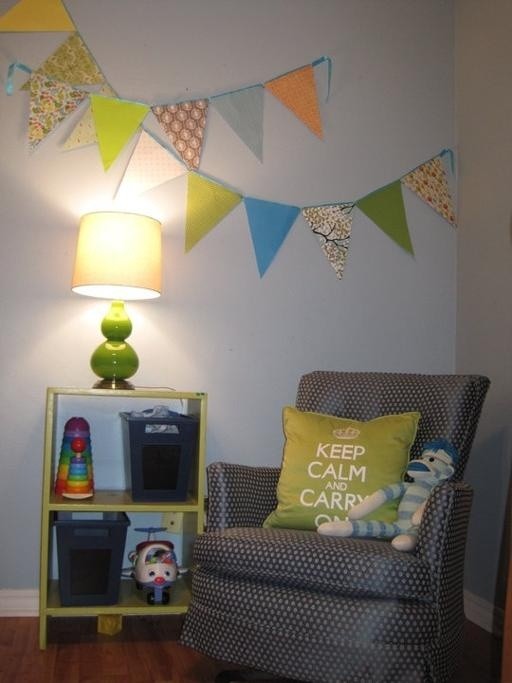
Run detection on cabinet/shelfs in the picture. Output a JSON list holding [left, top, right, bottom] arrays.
[[37, 385, 208, 650]]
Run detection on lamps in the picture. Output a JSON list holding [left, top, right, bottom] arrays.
[[70, 211, 163, 389]]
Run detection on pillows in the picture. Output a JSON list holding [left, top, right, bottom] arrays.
[[263, 406, 419, 531]]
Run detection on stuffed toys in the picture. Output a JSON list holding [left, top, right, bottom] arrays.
[[316, 441, 461, 550]]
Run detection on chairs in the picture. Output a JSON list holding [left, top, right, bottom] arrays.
[[176, 370, 492, 683]]
